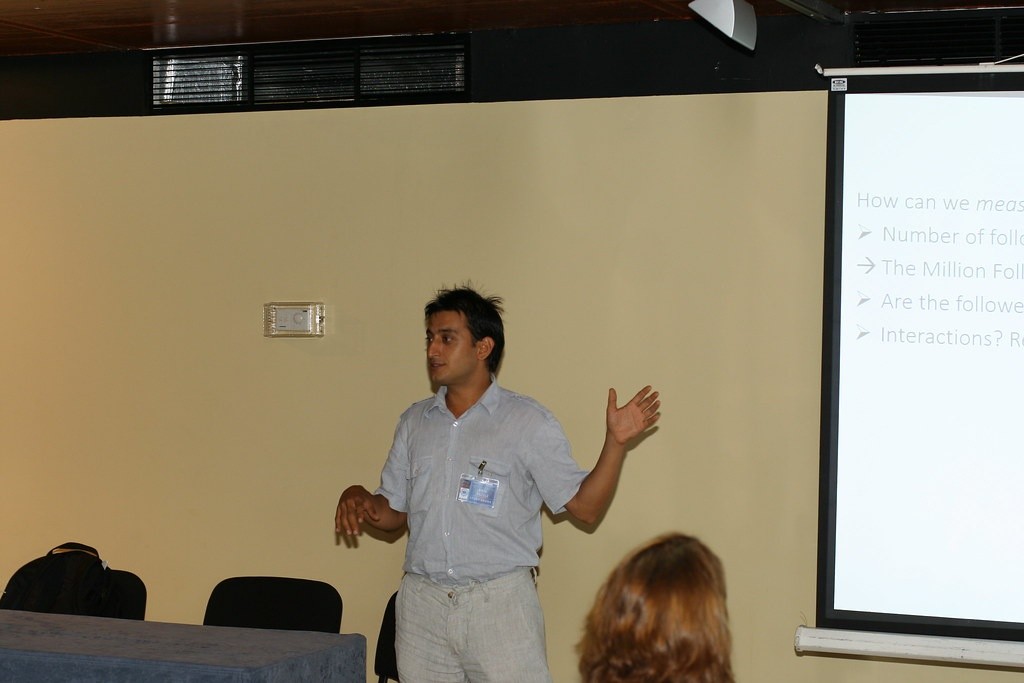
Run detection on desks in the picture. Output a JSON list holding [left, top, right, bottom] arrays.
[[2, 607, 370, 683]]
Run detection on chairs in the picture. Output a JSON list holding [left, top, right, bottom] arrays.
[[198, 578, 343, 633], [10, 564, 148, 621], [370, 591, 410, 682]]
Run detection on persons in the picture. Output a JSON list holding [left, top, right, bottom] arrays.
[[335, 283, 662, 681], [573, 530, 738, 682]]
